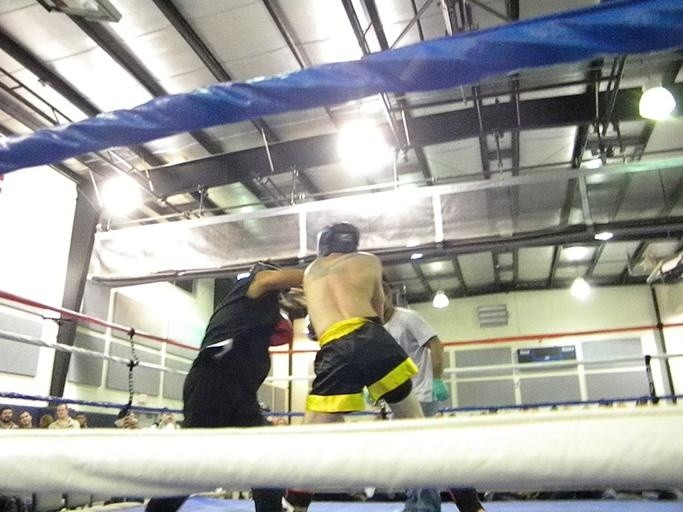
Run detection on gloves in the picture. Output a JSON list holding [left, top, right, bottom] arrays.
[[303, 314, 319, 342], [269, 311, 294, 347], [430, 379, 449, 401]]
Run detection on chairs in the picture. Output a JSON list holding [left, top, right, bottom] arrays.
[[91, 492, 113, 506], [61, 487, 90, 511], [28, 486, 64, 512]]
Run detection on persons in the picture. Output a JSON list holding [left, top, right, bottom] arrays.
[[283, 224, 482, 512], [379, 283, 444, 512], [146, 259, 303, 512]]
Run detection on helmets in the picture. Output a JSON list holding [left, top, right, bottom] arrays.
[[315, 222, 359, 260], [250, 259, 292, 294]]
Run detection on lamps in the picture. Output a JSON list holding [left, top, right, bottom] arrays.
[[566, 263, 595, 301], [428, 279, 453, 310], [633, 68, 678, 123]]
